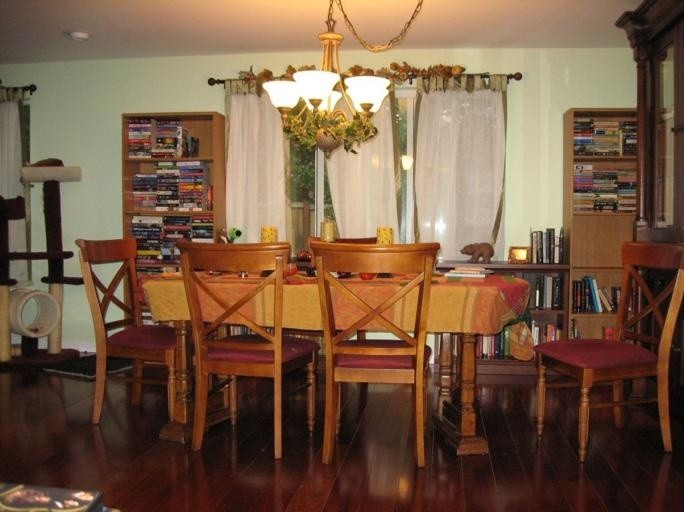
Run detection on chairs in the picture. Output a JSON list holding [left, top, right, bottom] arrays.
[[70, 236, 189, 425], [172, 236, 315, 459], [307, 239, 441, 468], [533, 239, 683, 468], [271, 229, 382, 408]]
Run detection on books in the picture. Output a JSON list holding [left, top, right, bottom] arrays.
[[123, 116, 215, 275]]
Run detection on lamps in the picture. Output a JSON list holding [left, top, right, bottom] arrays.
[[374, 225, 393, 246], [319, 221, 339, 244], [258, 0, 427, 153], [258, 223, 278, 245]]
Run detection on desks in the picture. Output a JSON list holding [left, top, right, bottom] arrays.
[[140, 267, 531, 460]]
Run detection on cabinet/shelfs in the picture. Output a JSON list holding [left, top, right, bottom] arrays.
[[614, 1, 683, 393], [436, 259, 569, 383], [561, 106, 641, 343], [115, 107, 228, 329]]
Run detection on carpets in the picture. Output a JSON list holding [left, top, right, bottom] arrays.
[[42, 350, 134, 380]]
